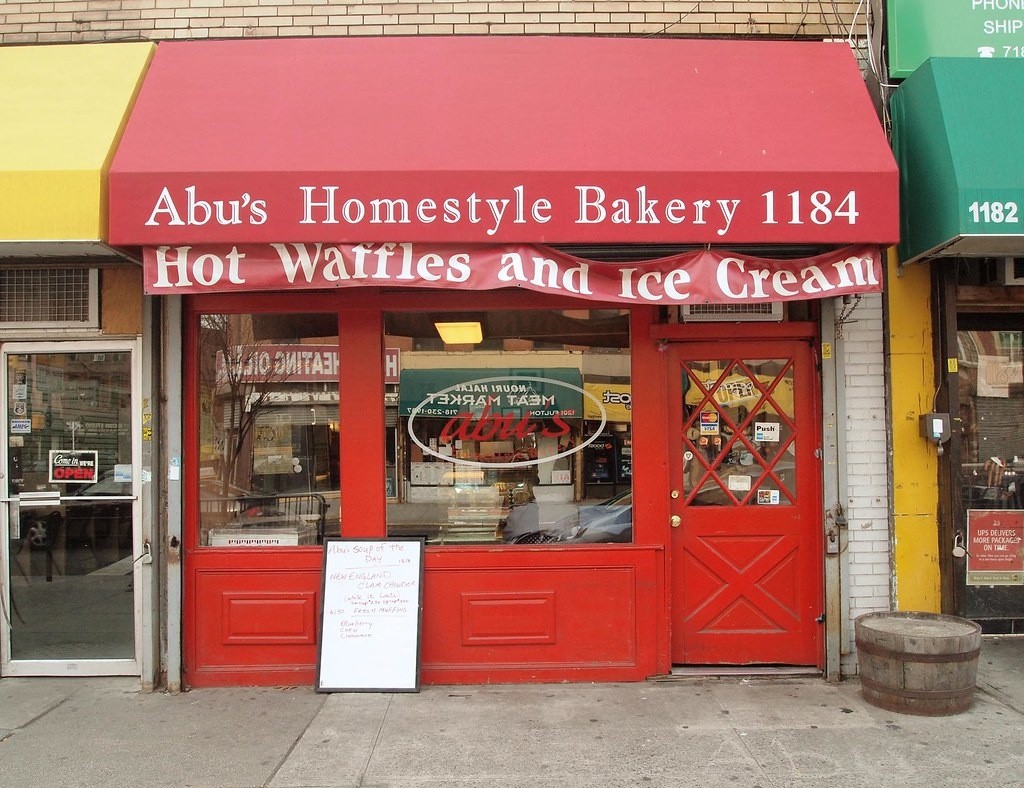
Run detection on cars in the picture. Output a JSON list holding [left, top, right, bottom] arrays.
[[20, 465, 280, 553], [506, 460, 798, 544]]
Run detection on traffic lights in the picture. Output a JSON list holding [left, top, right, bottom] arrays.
[[44, 410, 53, 428], [121, 394, 126, 408]]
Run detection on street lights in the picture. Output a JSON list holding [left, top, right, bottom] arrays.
[[71, 374, 85, 448]]
